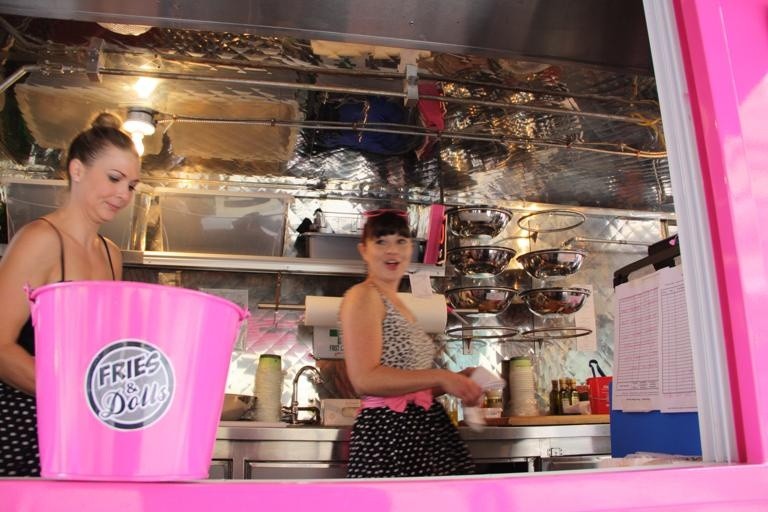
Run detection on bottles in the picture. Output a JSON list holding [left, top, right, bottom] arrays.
[[550, 379, 560, 415], [566, 379, 580, 406], [557, 378, 571, 416]]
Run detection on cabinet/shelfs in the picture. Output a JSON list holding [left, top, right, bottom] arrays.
[[0, 172, 458, 276], [444, 206, 592, 356]]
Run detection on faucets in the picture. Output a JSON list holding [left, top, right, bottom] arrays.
[[291, 365, 324, 406]]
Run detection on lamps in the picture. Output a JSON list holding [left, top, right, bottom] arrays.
[[122, 112, 156, 158]]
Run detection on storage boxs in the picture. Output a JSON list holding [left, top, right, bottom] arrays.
[[299, 231, 427, 263]]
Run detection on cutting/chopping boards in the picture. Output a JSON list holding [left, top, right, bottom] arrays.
[[484, 415, 611, 425]]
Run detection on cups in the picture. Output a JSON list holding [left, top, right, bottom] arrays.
[[509, 356, 538, 416], [256, 354, 282, 421]]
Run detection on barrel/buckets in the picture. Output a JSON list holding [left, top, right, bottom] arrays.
[[20, 281, 251, 483]]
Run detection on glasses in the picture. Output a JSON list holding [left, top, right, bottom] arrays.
[[364, 209, 408, 217]]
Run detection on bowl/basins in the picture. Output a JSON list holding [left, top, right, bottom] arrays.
[[443, 204, 513, 237], [222, 394, 256, 420], [444, 246, 517, 277], [461, 407, 503, 428], [515, 249, 586, 278], [518, 288, 592, 314], [441, 285, 517, 315]]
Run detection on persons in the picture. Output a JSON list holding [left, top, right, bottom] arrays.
[[339, 208, 483, 478], [1, 111, 141, 476]]
